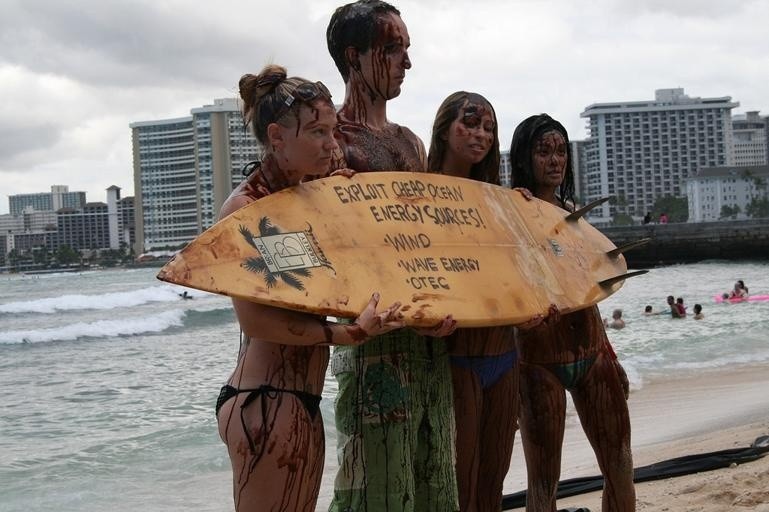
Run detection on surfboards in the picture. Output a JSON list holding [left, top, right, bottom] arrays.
[[157, 171, 650, 329]]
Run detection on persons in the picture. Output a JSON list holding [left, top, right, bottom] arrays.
[[644, 280, 704, 325], [215, 63, 404, 512], [321, 0, 460, 512], [659, 213, 667, 224], [644, 212, 655, 225], [609, 307, 624, 328], [427, 90, 561, 512], [511, 110, 638, 510], [721, 278, 749, 302]]
